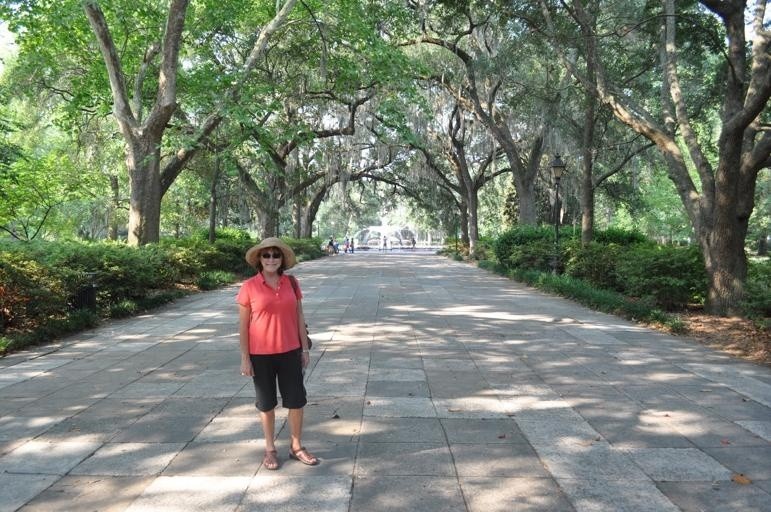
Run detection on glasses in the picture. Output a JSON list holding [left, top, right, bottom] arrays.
[[261, 253, 282, 258]]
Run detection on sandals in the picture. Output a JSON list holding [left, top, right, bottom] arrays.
[[288, 444, 317, 465], [264, 447, 279, 470]]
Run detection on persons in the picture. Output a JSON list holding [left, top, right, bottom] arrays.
[[383, 236, 387, 250], [235, 237, 319, 470], [329, 238, 354, 253], [412, 238, 416, 249]]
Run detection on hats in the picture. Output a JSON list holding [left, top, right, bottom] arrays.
[[245, 237, 296, 270]]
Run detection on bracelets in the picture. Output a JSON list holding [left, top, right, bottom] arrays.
[[301, 350, 309, 352]]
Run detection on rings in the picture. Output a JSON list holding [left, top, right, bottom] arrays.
[[242, 373, 245, 376]]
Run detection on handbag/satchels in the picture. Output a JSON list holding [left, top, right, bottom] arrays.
[[289, 274, 312, 349]]
